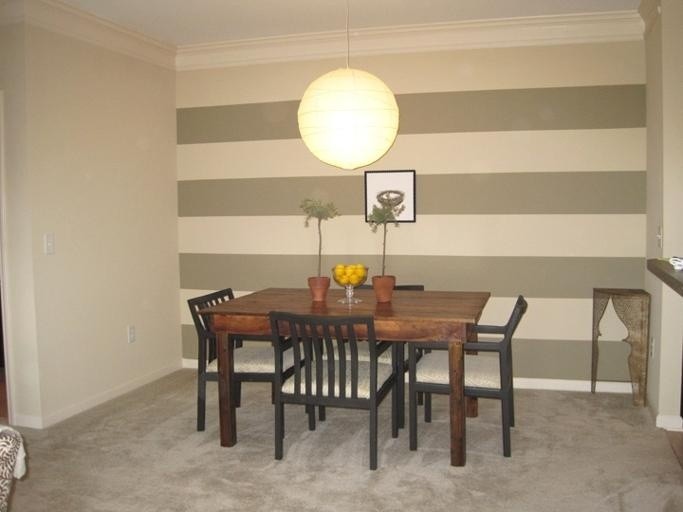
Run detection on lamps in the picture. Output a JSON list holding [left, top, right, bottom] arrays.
[[297, 0, 400, 170]]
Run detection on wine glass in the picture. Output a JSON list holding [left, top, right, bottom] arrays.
[[332, 267, 368, 304]]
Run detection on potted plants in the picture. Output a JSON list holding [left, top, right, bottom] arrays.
[[299, 195, 342, 301], [368, 203, 398, 303]]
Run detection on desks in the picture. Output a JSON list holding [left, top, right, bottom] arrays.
[[591, 289, 651, 408], [197, 287, 489, 468]]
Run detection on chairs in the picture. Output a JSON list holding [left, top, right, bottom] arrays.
[[269, 311, 399, 469], [319, 285, 426, 430], [188, 287, 312, 439], [410, 294, 528, 458]]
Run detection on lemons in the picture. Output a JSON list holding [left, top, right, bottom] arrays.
[[333, 263, 366, 288]]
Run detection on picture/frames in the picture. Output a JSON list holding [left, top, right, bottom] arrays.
[[364, 169, 416, 223]]
